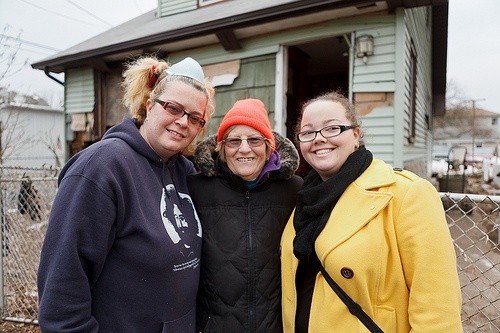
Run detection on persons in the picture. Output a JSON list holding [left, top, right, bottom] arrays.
[[185, 99, 304, 333], [280, 92, 462, 333], [37, 55, 216, 333]]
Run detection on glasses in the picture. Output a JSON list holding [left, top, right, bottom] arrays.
[[222, 136, 264, 149], [154, 99, 206, 129], [296, 125, 356, 143]]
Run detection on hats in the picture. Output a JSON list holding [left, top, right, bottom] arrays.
[[218, 98, 276, 151]]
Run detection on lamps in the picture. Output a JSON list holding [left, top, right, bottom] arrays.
[[355, 34, 375, 63]]
